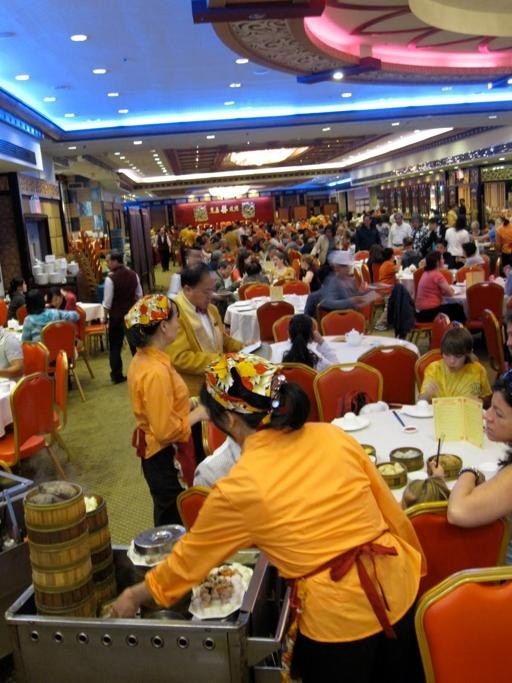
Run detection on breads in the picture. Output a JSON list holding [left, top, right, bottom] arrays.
[[29, 481, 78, 506]]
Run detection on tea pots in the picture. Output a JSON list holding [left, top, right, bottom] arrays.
[[344, 327, 364, 346]]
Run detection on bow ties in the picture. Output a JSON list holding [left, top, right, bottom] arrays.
[[196, 306, 207, 313]]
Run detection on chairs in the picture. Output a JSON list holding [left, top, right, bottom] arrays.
[[0, 221, 512, 682]]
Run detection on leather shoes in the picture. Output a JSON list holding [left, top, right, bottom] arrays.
[[112, 376, 127, 383]]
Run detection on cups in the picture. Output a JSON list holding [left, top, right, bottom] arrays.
[[1, 378, 10, 393], [415, 399, 430, 413], [343, 412, 358, 426]]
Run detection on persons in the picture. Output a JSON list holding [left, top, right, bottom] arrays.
[[1, 199, 512, 682]]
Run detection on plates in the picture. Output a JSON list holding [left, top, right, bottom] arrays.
[[32, 253, 80, 285], [236, 306, 255, 311], [339, 415, 370, 431], [234, 301, 251, 306], [403, 408, 433, 418], [126, 538, 169, 568], [191, 561, 254, 619]]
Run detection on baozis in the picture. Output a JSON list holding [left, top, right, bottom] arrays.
[[392, 449, 419, 459], [377, 463, 404, 476], [434, 454, 460, 467], [83, 495, 99, 513]]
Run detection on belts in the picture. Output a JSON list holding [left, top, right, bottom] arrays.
[[394, 244, 402, 246]]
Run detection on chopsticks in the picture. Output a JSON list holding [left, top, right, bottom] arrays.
[[392, 410, 405, 427], [435, 437, 441, 470]]
